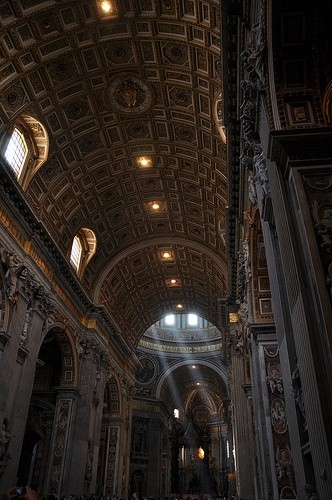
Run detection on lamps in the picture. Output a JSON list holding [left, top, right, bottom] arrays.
[[134, 155, 155, 169], [147, 200, 166, 213], [168, 278, 180, 286], [96, 0, 118, 20], [175, 304, 184, 309], [160, 251, 172, 258]]
